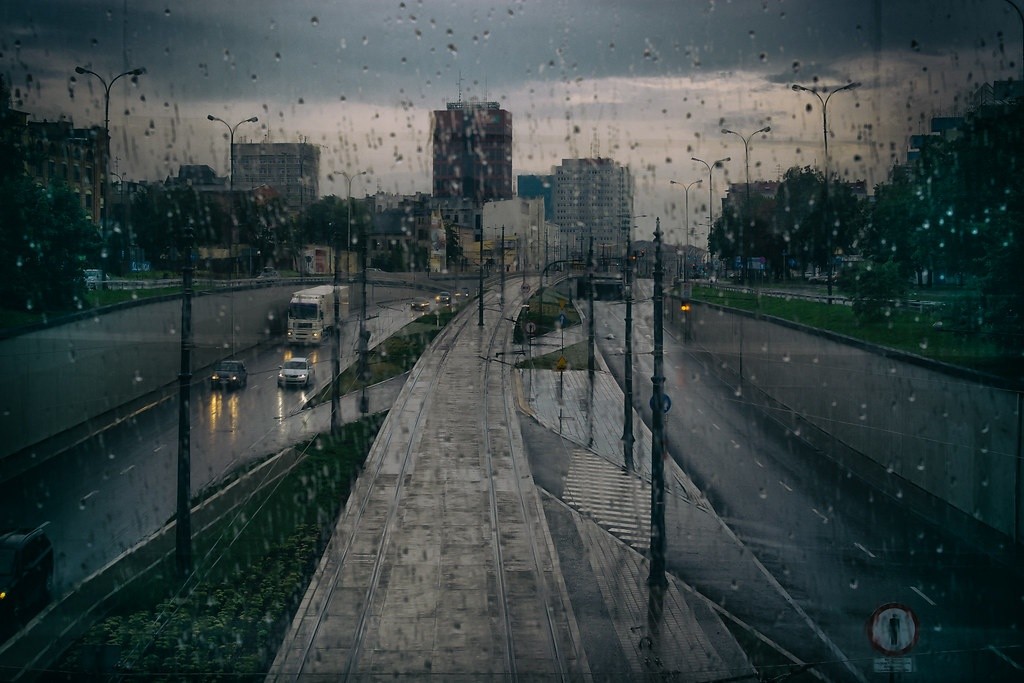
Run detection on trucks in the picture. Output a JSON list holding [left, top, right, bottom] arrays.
[[287, 285, 350, 346]]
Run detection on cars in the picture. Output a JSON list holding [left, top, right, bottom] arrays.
[[211, 361, 248, 389], [277, 357, 315, 386], [410, 297, 429, 312], [816, 272, 837, 282], [729, 272, 740, 279], [82, 269, 113, 291], [0, 527, 54, 618], [804, 272, 818, 278], [436, 292, 451, 304]]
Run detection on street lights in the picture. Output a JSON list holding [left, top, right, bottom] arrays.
[[691, 157, 730, 264], [602, 242, 605, 271], [249, 248, 261, 276], [617, 215, 647, 283], [76, 66, 143, 290], [722, 126, 771, 287], [334, 171, 366, 279], [670, 180, 702, 246], [281, 152, 322, 281], [793, 82, 862, 306], [207, 115, 258, 279], [554, 241, 556, 261]]
